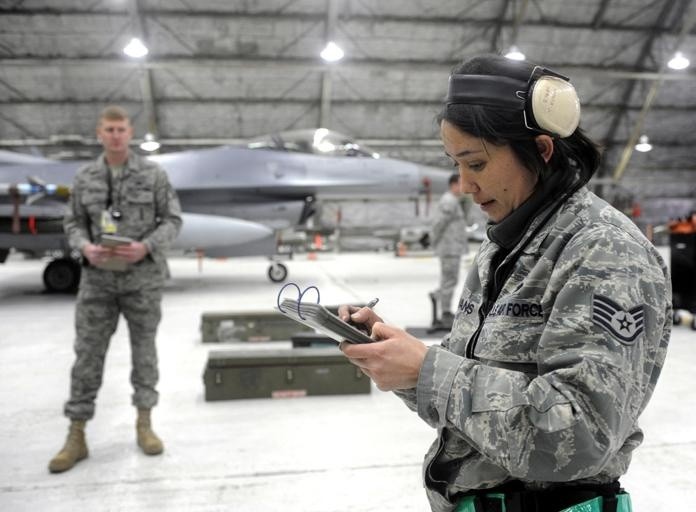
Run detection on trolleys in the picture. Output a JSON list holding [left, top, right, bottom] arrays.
[[664, 212, 695, 331]]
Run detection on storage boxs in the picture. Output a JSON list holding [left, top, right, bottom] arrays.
[[203, 349, 371, 401], [200, 305, 366, 344]]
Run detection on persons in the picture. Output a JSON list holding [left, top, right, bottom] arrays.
[[430, 173, 470, 326], [48, 104, 184, 474], [334, 53, 674, 512]]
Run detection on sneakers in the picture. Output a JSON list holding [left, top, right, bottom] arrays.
[[441, 312, 456, 321]]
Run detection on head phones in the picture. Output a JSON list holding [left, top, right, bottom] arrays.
[[448, 66, 580, 139]]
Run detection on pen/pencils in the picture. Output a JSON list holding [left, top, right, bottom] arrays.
[[348, 298, 379, 324]]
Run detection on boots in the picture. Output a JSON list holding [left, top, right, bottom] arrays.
[[136, 408, 163, 455], [49, 419, 89, 473]]
